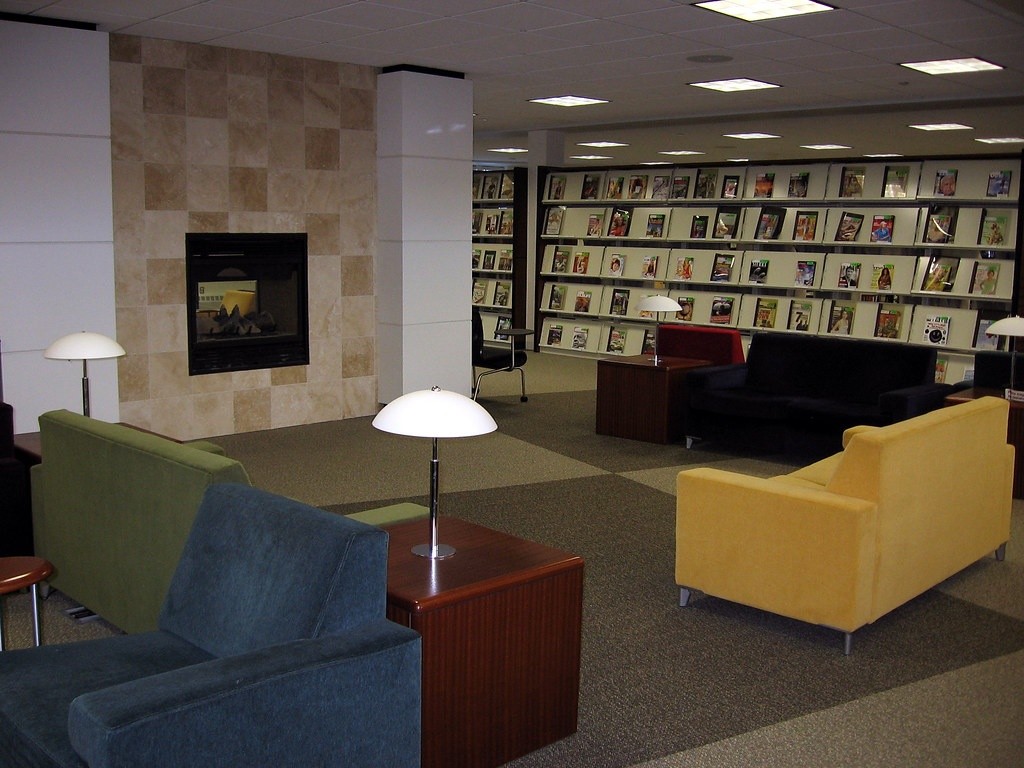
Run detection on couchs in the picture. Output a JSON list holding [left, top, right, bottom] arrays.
[[33, 410, 430, 637], [675, 394, 1015, 652], [669, 330, 952, 455], [0, 480, 422, 768]]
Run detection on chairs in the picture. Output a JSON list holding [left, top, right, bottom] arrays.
[[655, 323, 745, 366], [973, 350, 1024, 392]]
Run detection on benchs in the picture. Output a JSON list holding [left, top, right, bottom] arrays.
[[472, 306, 529, 403]]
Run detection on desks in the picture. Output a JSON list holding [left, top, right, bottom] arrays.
[[494, 329, 535, 371], [382, 517, 584, 768], [596, 354, 711, 444], [944, 387, 1024, 500]]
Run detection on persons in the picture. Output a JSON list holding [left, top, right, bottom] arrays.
[[980, 269, 996, 296], [831, 309, 849, 335], [682, 258, 692, 279], [554, 181, 561, 200], [488, 178, 495, 199], [610, 258, 622, 275], [939, 175, 956, 196], [841, 266, 856, 289], [576, 256, 588, 273], [877, 268, 891, 290], [724, 181, 734, 195], [871, 221, 891, 242], [644, 260, 654, 276], [573, 332, 585, 349]]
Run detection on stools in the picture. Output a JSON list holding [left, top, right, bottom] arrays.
[[0, 555, 53, 648]]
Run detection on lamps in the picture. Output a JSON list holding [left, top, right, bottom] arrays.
[[639, 294, 683, 362], [985, 315, 1024, 397], [45, 331, 125, 418], [371, 385, 498, 558]]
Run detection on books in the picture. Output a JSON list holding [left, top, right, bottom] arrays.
[[471, 172, 514, 341], [546, 168, 1011, 383]]
